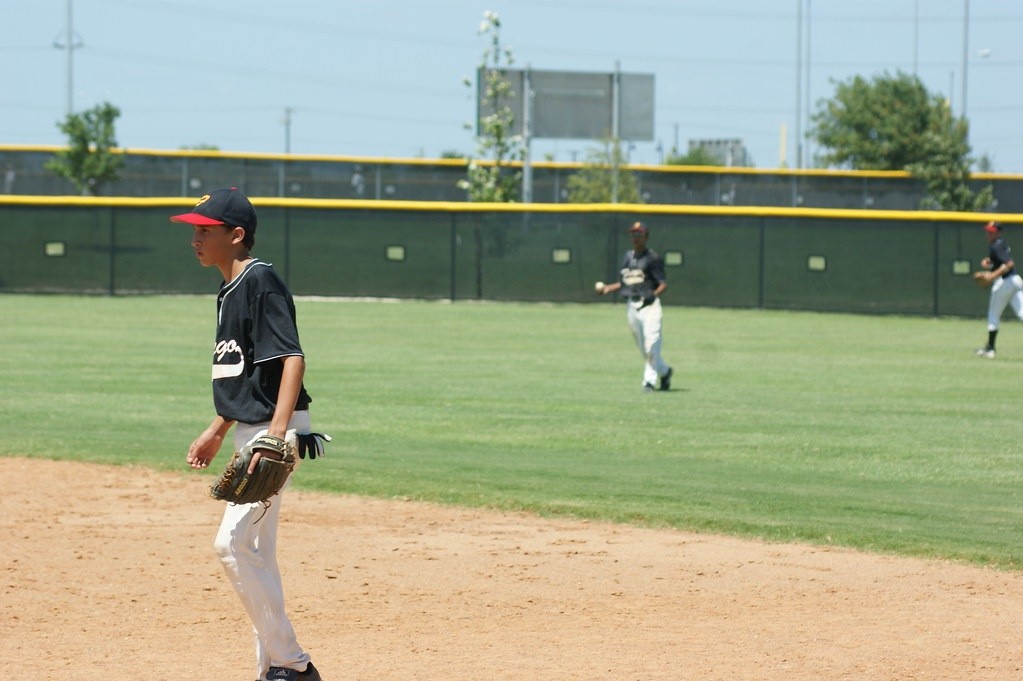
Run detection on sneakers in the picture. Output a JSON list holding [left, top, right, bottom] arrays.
[[975, 348, 997, 359], [641, 380, 655, 391], [660, 368, 674, 391], [254, 662, 323, 681]]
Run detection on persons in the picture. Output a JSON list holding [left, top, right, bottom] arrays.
[[595, 220, 673, 390], [168, 188, 332, 680], [973, 221, 1023, 359]]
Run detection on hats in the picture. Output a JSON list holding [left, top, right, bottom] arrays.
[[170, 187, 257, 233], [984, 221, 1003, 233], [628, 220, 649, 232]]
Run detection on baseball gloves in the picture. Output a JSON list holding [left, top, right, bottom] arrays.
[[628, 294, 655, 311], [974, 271, 993, 289], [206, 434, 297, 526]]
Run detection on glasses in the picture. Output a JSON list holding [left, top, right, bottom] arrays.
[[629, 232, 646, 237]]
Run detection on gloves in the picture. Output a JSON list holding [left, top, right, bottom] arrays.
[[296, 432, 332, 459]]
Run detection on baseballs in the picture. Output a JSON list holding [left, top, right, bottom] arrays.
[[595, 281, 604, 292]]
